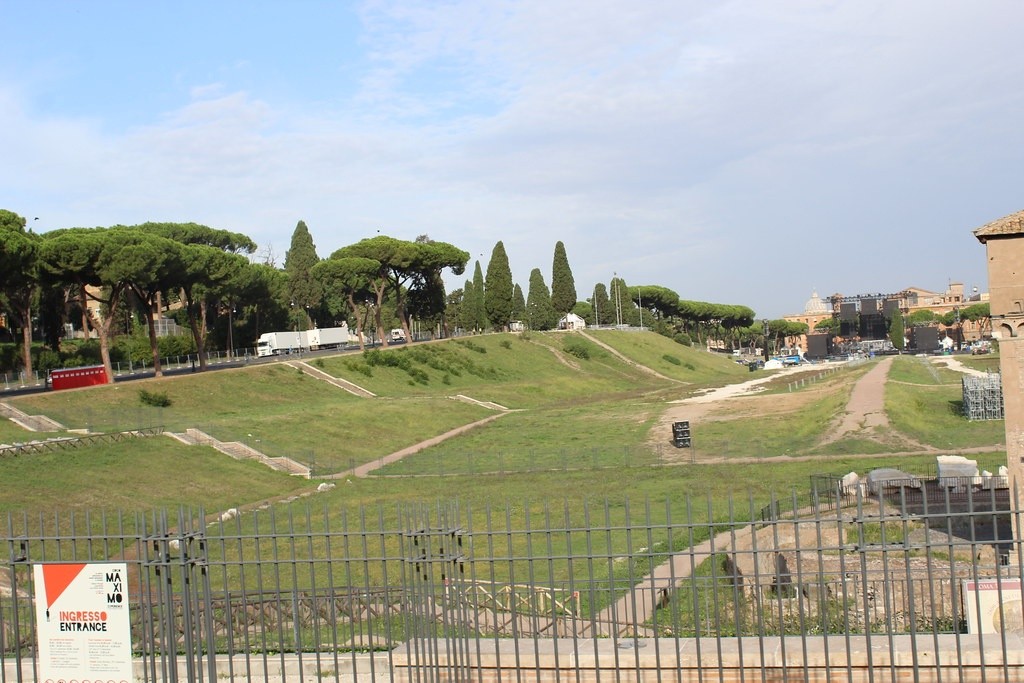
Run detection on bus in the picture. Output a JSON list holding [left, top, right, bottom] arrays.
[[770, 355, 801, 366]]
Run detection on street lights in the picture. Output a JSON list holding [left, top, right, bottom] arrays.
[[228, 304, 236, 359], [305, 298, 311, 330], [452, 300, 458, 336], [125, 310, 135, 375], [288, 298, 301, 357]]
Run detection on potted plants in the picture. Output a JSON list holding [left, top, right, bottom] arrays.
[[765, 553, 799, 615]]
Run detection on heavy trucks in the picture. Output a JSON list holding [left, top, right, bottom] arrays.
[[256, 326, 350, 358]]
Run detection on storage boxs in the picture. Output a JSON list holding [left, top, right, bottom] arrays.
[[673, 420, 691, 448]]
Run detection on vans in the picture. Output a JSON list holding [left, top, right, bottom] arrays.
[[391, 329, 412, 342]]
